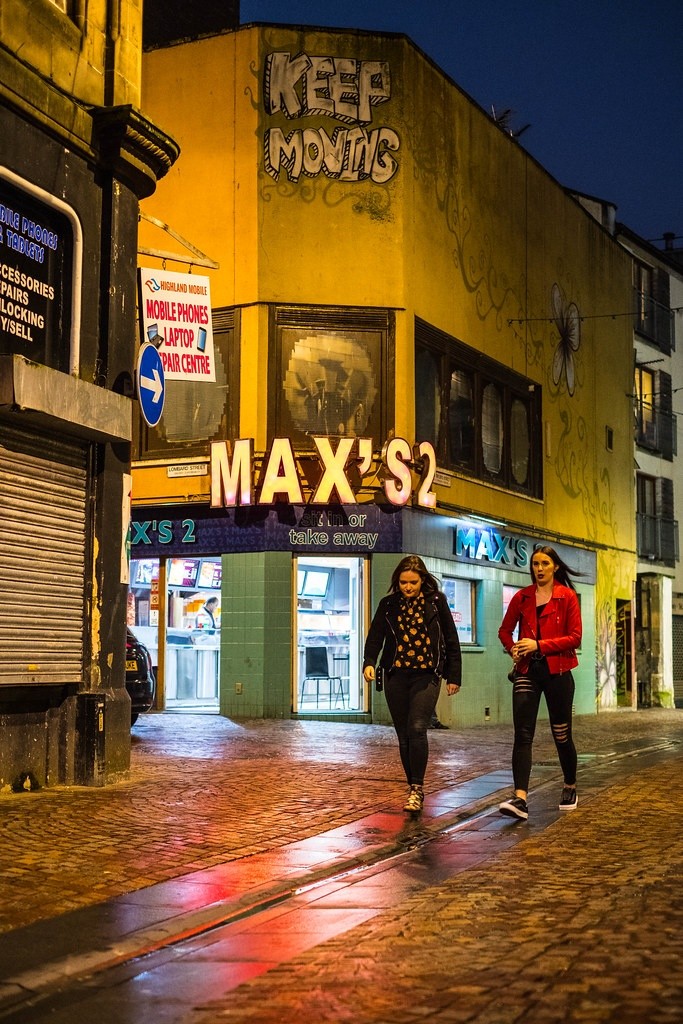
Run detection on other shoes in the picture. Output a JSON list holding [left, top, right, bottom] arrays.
[[402, 786, 425, 812]]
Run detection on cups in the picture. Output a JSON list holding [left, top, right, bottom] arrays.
[[516, 647, 529, 675]]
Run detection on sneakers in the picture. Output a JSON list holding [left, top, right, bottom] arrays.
[[559, 786, 579, 811], [499, 791, 529, 820]]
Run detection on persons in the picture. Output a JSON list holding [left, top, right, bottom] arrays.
[[362, 555, 462, 811], [195, 597, 219, 628], [497, 545, 582, 820]]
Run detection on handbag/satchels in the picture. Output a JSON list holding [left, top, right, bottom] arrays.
[[507, 662, 516, 684]]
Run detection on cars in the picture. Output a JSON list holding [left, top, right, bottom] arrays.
[[126, 623, 154, 725]]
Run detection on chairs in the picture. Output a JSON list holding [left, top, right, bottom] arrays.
[[300, 646, 344, 709]]
